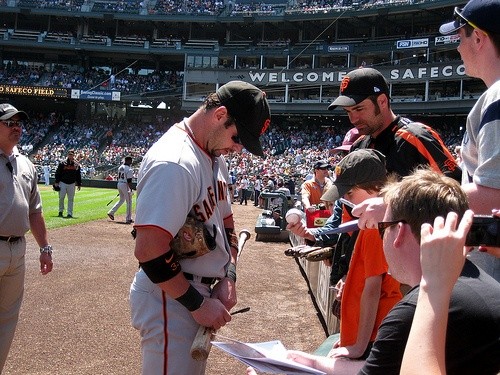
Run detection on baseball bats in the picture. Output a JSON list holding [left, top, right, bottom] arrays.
[[188, 230, 251, 362], [106, 193, 119, 206]]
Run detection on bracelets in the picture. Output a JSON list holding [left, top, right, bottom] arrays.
[[174, 285, 204, 312]]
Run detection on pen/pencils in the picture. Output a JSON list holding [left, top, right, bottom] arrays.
[[231, 307, 249, 315]]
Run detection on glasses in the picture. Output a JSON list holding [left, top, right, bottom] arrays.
[[0, 119, 23, 127], [229, 126, 243, 145], [68, 153, 73, 155], [453, 7, 488, 36], [378, 220, 410, 239]]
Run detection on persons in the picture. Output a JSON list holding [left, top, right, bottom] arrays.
[[13, 109, 290, 207], [107, 157, 135, 223], [285, 0, 500, 375], [129, 80, 270, 375], [54, 149, 82, 218], [0, 103, 53, 375], [0, 0, 298, 103]]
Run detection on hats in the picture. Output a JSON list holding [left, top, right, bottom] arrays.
[[216, 81, 271, 159], [330, 127, 362, 152], [328, 65, 389, 111], [320, 148, 387, 201], [0, 103, 29, 123], [68, 149, 74, 153], [439, 0, 500, 36], [314, 160, 331, 169]]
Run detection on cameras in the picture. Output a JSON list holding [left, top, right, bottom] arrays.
[[466, 214, 500, 246]]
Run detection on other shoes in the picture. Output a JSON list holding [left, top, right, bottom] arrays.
[[126, 220, 134, 223], [108, 214, 114, 220], [59, 212, 63, 217], [67, 214, 73, 218]]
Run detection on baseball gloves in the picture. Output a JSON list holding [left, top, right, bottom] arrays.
[[53, 184, 61, 192]]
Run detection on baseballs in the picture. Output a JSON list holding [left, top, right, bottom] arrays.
[[285, 208, 302, 226]]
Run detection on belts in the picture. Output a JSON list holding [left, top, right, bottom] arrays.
[[183, 272, 217, 285], [0, 236, 21, 242]]
